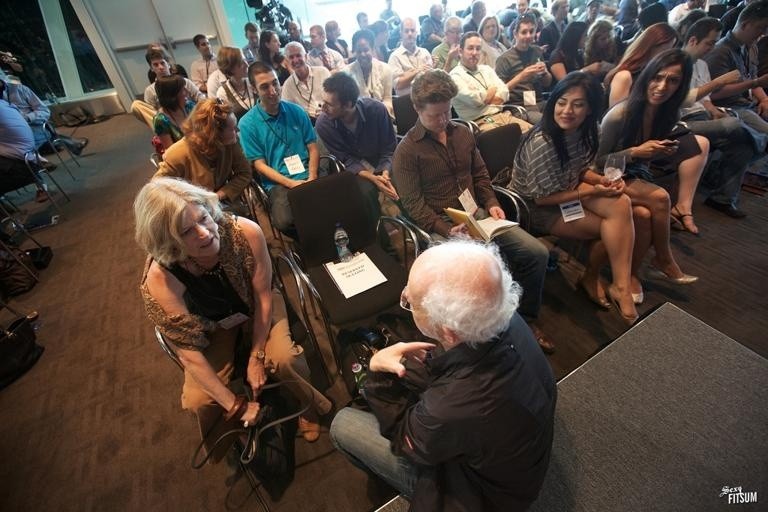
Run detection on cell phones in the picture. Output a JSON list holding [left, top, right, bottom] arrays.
[[661, 141, 678, 147]]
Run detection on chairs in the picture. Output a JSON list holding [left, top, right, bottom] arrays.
[[145, 0, 767, 511], [0, 73, 79, 322]]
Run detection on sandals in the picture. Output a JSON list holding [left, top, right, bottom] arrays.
[[297, 410, 322, 441], [670, 205, 699, 234], [607, 283, 639, 326], [589, 282, 613, 308]]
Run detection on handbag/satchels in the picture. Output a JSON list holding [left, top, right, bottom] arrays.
[[0, 314, 46, 395], [236, 381, 300, 499], [0, 236, 40, 299], [58, 106, 92, 127]]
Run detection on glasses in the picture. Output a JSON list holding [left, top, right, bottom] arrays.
[[400, 286, 416, 313]]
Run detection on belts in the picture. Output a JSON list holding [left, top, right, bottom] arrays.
[[470, 111, 503, 122]]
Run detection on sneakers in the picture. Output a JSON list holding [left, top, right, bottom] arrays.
[[37, 184, 49, 202]]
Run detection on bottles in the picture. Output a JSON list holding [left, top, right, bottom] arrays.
[[604, 154, 626, 182], [351, 364, 369, 402], [44, 93, 60, 104], [335, 223, 354, 263]]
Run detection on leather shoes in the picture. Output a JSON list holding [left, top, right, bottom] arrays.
[[704, 196, 748, 218], [629, 285, 644, 304], [527, 323, 557, 353], [643, 265, 698, 286]]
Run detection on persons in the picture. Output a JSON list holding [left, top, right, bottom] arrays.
[[131, 0, 768, 512], [0, 42, 90, 244]]
